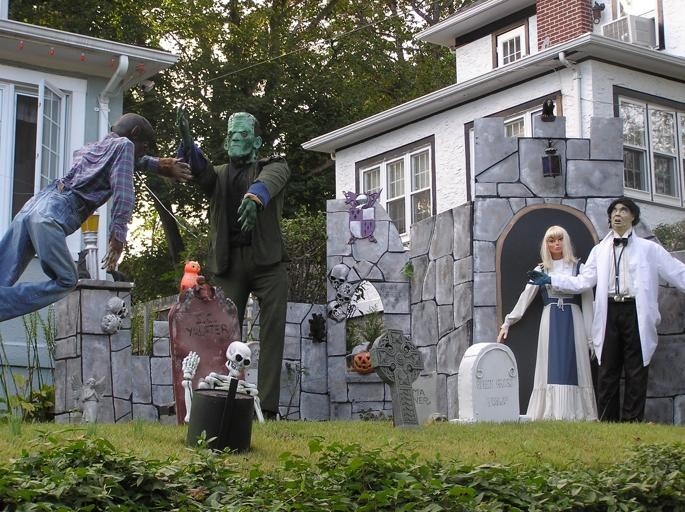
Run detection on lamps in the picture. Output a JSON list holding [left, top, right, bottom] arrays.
[[591, 1, 606, 24], [542, 141, 564, 179]]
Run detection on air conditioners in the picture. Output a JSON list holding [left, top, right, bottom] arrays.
[[600, 16, 655, 48]]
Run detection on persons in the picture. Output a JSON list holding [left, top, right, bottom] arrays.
[[0, 113, 191, 326], [176, 98, 290, 420], [498, 226, 600, 421], [526, 197, 685, 421]]
